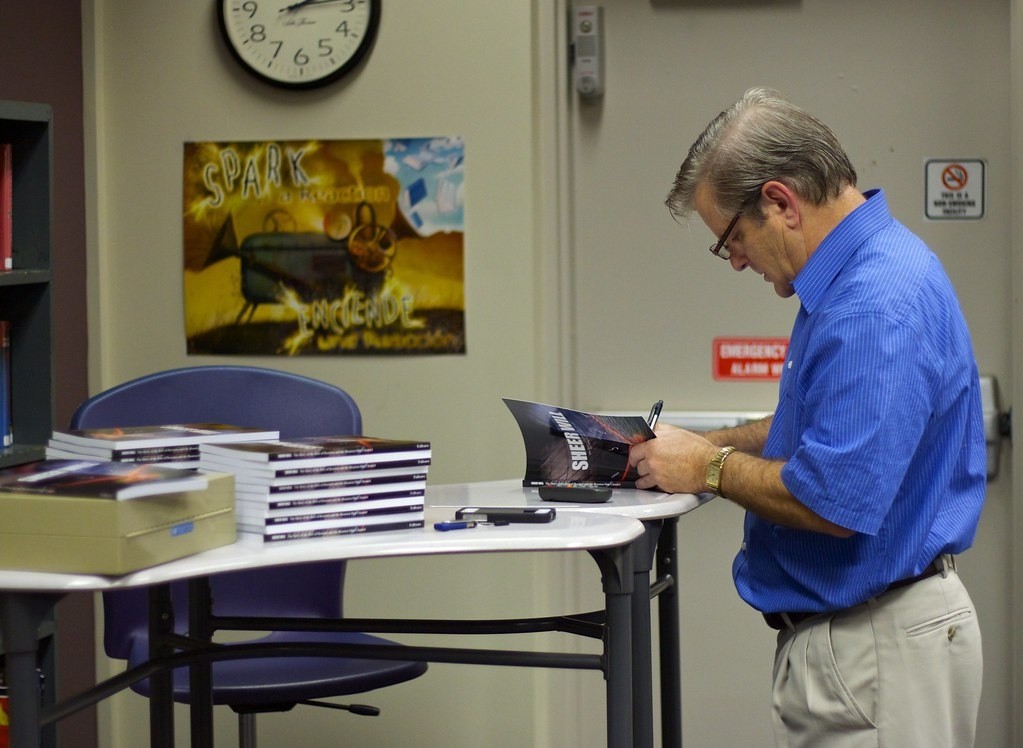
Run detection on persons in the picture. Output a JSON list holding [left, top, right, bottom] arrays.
[[625, 95, 988, 748]]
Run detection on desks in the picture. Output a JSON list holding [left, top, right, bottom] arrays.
[[425, 479, 718, 748], [0, 505, 649, 748]]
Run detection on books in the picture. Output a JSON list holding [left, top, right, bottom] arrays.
[[0, 138, 13, 270], [195, 434, 432, 547], [501, 395, 664, 494], [1, 316, 14, 448], [43, 421, 281, 473]]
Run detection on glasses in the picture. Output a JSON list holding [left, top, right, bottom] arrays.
[[709, 199, 748, 261]]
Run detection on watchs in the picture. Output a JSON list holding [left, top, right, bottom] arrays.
[[705, 445, 737, 499]]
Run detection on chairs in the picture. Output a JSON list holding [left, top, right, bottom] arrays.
[[69, 366, 426, 748]]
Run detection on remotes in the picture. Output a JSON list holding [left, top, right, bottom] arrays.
[[539, 482, 612, 503]]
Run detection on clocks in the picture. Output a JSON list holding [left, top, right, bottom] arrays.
[[215, 0, 382, 92]]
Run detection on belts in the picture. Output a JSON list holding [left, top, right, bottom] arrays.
[[763, 554, 945, 629]]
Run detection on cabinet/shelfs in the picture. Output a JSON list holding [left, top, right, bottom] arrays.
[[1, 99, 58, 469]]
